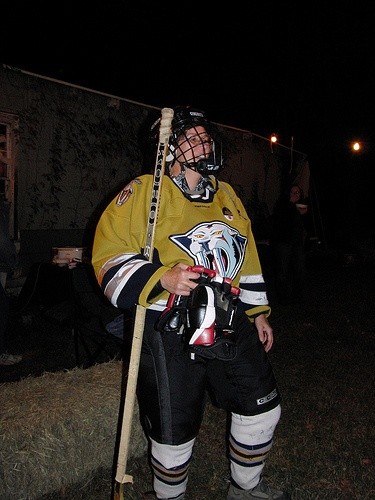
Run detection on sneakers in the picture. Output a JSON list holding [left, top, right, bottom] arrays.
[[227, 477, 291, 500]]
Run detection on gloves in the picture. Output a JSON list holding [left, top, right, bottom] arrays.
[[156, 266, 240, 360]]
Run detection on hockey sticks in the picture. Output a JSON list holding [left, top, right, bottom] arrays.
[[112, 105, 173, 499]]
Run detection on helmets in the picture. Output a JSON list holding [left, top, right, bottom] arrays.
[[149, 105, 211, 162]]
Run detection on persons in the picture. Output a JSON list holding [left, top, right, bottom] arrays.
[[0, 217, 23, 366], [273, 184, 307, 304], [92, 104, 288, 500]]
